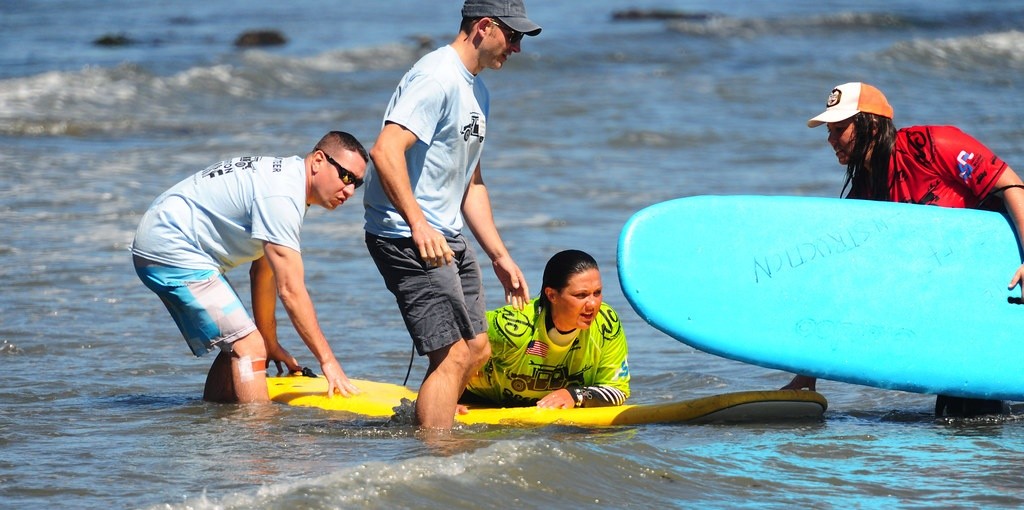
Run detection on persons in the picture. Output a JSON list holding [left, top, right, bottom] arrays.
[[364, 0, 543, 428], [134, 131, 357, 403], [457, 250, 630, 410], [779, 82, 1024, 420]]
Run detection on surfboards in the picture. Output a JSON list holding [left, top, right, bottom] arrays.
[[267, 374, 829, 432], [616, 193, 1024, 396]]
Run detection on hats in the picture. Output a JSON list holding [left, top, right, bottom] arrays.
[[461, 0, 542, 37], [807, 82, 893, 128]]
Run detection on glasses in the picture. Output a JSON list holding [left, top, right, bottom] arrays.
[[489, 19, 524, 44], [315, 148, 364, 189]]
